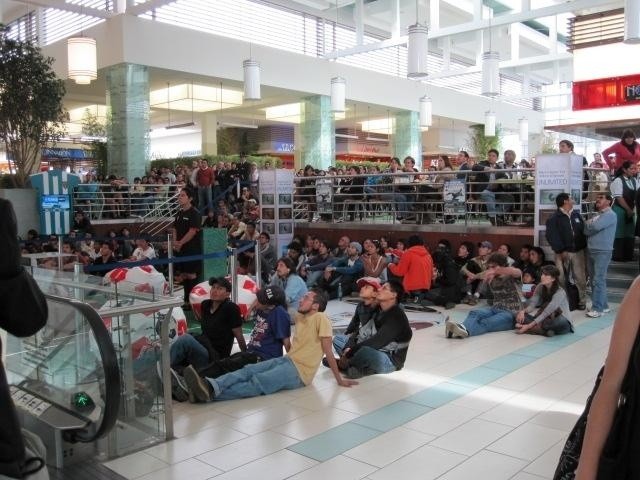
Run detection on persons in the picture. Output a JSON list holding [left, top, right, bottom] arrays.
[[352, 279, 416, 377], [117, 277, 248, 373], [442, 252, 526, 341], [582, 189, 615, 317], [545, 194, 588, 312], [77, 128, 640, 303], [516, 263, 577, 337], [2, 200, 49, 479], [20, 208, 76, 283], [167, 287, 292, 401], [212, 289, 343, 403], [327, 273, 383, 368]]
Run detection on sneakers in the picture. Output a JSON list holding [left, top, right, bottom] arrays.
[[413, 293, 425, 303], [322, 358, 340, 369], [463, 294, 472, 304], [545, 330, 557, 336], [446, 302, 455, 309], [587, 311, 601, 318], [347, 365, 375, 379], [444, 217, 455, 224], [603, 309, 610, 312], [577, 304, 587, 310], [445, 316, 453, 337], [446, 320, 469, 337], [183, 367, 210, 403], [170, 368, 189, 401], [469, 294, 480, 305]]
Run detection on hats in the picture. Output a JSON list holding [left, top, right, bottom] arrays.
[[350, 242, 363, 255], [247, 199, 257, 204], [356, 276, 382, 288], [134, 177, 142, 181], [409, 235, 423, 244], [476, 241, 492, 248], [209, 276, 232, 292], [239, 151, 248, 157], [256, 286, 285, 305]]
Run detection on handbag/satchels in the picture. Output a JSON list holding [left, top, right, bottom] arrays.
[[566, 283, 578, 311], [95, 365, 154, 416], [543, 314, 571, 334], [356, 319, 398, 353]]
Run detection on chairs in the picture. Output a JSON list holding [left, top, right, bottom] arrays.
[[154, 184, 172, 217], [298, 176, 486, 222]]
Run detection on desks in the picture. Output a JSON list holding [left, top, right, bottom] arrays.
[[493, 178, 535, 225]]
